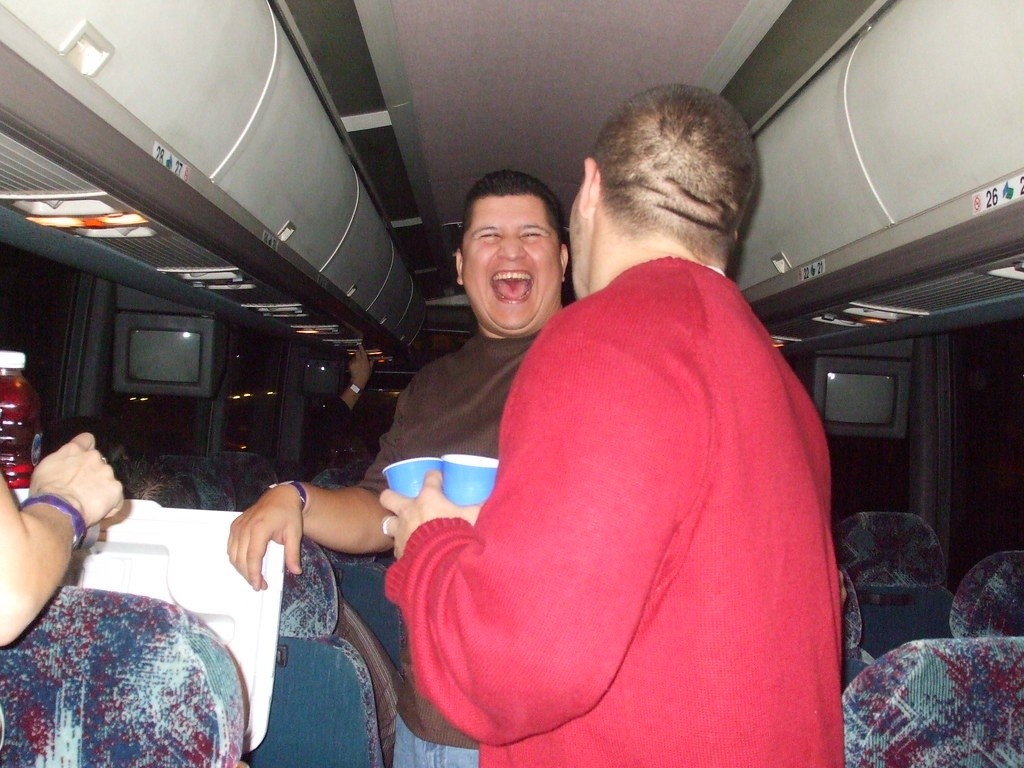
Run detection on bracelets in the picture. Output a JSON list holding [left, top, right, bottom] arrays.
[[346, 382, 362, 396], [268, 480, 306, 511], [22, 494, 87, 553]]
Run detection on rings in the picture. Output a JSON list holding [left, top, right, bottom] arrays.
[[100, 456, 111, 464], [381, 513, 394, 539]]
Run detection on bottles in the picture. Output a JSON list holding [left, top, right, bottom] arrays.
[[0, 350, 43, 489]]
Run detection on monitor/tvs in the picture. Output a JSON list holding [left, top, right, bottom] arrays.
[[113, 313, 229, 400], [303, 359, 345, 399], [812, 356, 909, 439]]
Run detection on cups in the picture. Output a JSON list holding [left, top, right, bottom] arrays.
[[440, 454, 499, 506], [382, 458, 444, 498]]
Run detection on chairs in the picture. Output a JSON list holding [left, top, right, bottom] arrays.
[[1, 455, 1024, 767]]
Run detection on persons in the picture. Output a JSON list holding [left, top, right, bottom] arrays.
[[0, 84, 849, 768]]
